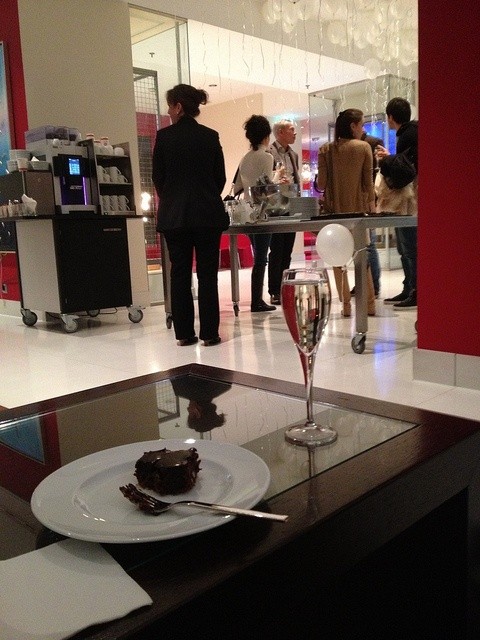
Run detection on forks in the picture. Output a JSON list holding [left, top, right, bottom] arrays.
[[120, 483, 289, 525]]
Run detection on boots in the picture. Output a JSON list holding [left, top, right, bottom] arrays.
[[333, 265, 351, 318], [367, 265, 376, 316]]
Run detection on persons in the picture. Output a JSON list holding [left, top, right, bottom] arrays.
[[348, 122, 386, 298], [232, 115, 276, 314], [267, 119, 300, 302], [312, 109, 376, 317], [374, 97, 419, 309], [151, 84, 230, 347]]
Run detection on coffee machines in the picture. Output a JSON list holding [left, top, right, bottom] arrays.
[[43, 125, 99, 213]]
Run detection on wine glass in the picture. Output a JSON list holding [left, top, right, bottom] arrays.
[[280, 268, 337, 446]]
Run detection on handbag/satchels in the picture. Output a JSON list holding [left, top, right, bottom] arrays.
[[315, 200, 331, 215], [223, 166, 244, 201]]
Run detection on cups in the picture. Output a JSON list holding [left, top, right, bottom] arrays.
[[114, 146, 124, 156], [17, 157, 28, 172], [6, 161, 17, 172], [99, 195, 130, 212], [97, 166, 128, 182]]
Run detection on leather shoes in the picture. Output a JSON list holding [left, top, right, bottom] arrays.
[[271, 292, 281, 304], [204, 336, 221, 346], [177, 336, 198, 346], [251, 304, 276, 312]]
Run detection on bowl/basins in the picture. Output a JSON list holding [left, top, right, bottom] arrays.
[[247, 183, 301, 217]]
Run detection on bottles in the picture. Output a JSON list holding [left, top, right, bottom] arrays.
[[84, 133, 113, 156], [0, 199, 37, 217]]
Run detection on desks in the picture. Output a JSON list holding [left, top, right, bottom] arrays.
[[156, 216, 418, 354]]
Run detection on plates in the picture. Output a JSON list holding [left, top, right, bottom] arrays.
[[30, 437, 271, 545], [289, 196, 317, 220]]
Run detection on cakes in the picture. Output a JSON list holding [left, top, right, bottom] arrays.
[[133, 447, 203, 497]]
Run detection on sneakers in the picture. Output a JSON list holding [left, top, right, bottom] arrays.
[[383, 291, 407, 303], [393, 296, 418, 311]]
